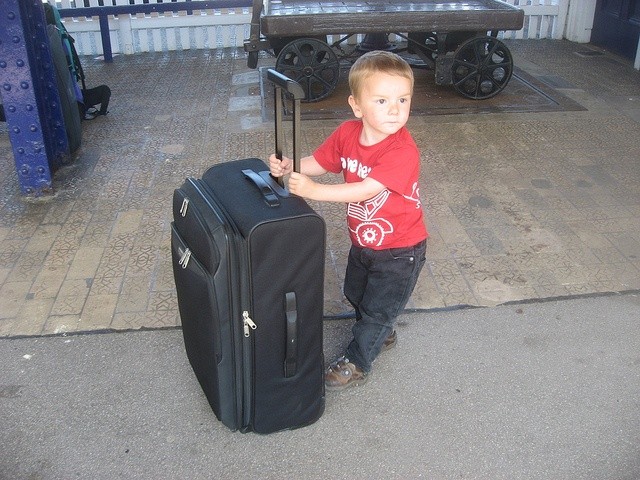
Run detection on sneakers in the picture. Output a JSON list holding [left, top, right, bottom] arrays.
[[379, 331, 397, 355], [324, 356, 368, 391]]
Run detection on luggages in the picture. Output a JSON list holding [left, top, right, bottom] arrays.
[[170, 68, 327, 436]]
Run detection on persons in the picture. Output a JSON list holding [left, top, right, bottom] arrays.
[[270, 49, 429, 391]]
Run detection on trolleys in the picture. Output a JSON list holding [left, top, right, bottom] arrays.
[[241, 0, 524, 103]]
